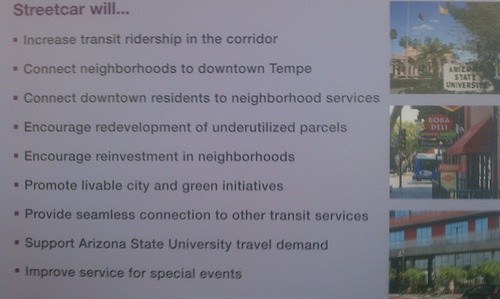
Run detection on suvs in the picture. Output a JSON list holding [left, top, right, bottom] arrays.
[[462, 284, 500, 299]]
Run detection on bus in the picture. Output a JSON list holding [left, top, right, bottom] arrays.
[[414, 154, 443, 182]]
